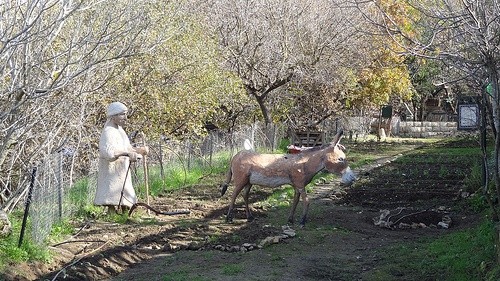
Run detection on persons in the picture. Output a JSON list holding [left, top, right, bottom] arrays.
[[95, 101, 150, 217]]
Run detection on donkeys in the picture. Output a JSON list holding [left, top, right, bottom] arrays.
[[215, 129, 356, 226]]
